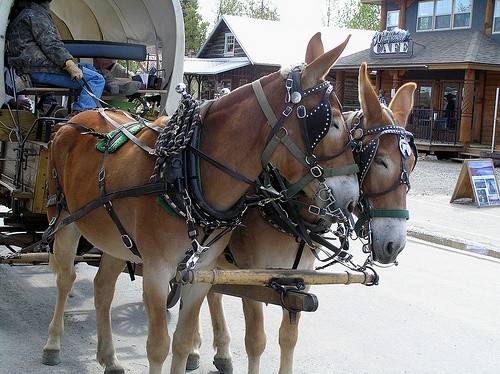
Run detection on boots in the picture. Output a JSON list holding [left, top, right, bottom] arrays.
[[66, 111, 80, 119], [98, 69, 141, 95]]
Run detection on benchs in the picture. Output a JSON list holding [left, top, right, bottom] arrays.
[[136, 90, 167, 115], [17, 87, 73, 143]]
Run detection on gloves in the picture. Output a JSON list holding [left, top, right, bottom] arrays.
[[8, 99, 31, 110], [66, 60, 83, 82]]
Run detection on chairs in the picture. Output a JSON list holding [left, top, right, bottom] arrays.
[[412, 109, 460, 145]]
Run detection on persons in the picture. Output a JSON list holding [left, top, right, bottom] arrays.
[[3, 0, 142, 122], [377, 89, 388, 108], [92, 57, 136, 81]]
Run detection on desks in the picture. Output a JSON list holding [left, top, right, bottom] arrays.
[[419, 119, 445, 142]]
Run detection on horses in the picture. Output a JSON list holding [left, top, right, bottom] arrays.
[[39, 30, 420, 374]]
